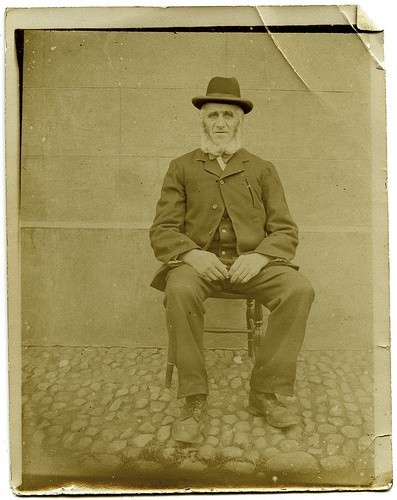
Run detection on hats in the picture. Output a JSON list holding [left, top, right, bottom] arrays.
[[191, 76, 254, 114]]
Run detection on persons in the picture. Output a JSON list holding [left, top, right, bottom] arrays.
[[149, 76, 315, 444]]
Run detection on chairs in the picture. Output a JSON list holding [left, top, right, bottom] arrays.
[[164, 291, 263, 389]]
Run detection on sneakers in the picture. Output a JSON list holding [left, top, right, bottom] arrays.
[[172, 395, 210, 443], [248, 389, 300, 428]]
